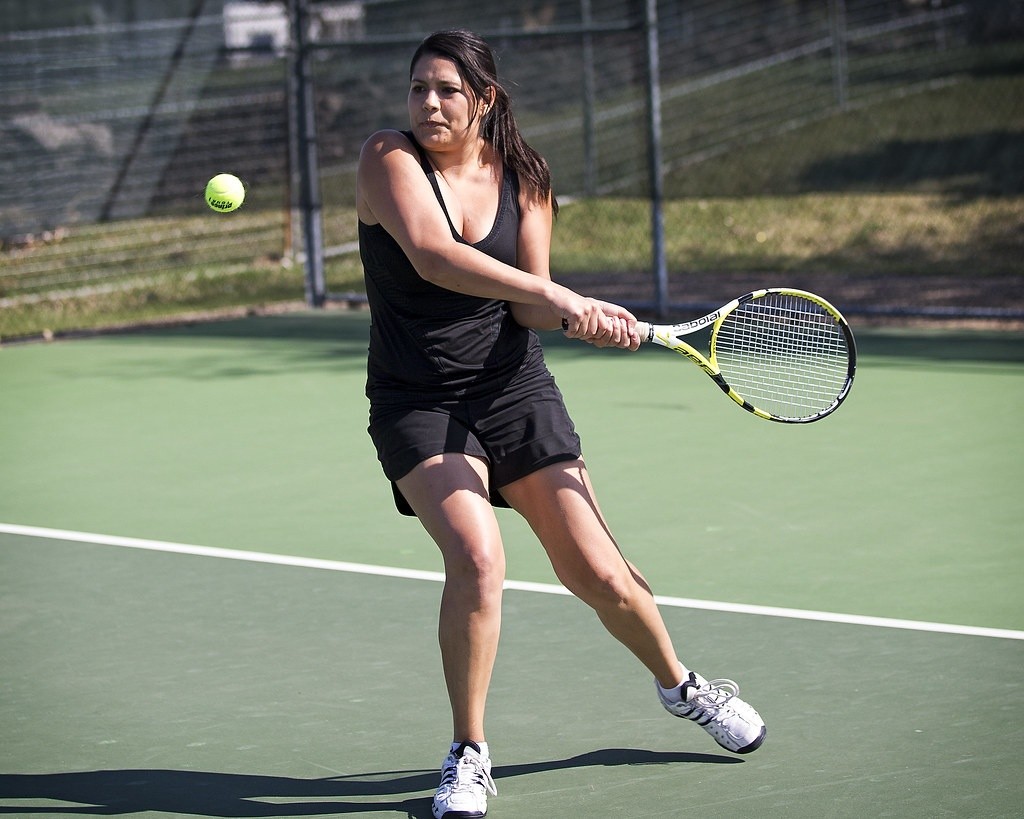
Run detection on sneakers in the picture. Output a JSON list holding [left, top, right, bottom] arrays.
[[432, 738, 498, 819], [654, 662, 767, 754]]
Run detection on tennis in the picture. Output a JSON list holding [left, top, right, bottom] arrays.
[[203, 173, 244, 213]]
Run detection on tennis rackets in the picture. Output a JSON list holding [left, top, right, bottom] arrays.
[[563, 285, 860, 425]]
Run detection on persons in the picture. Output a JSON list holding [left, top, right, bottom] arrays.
[[356, 30, 766, 819]]
